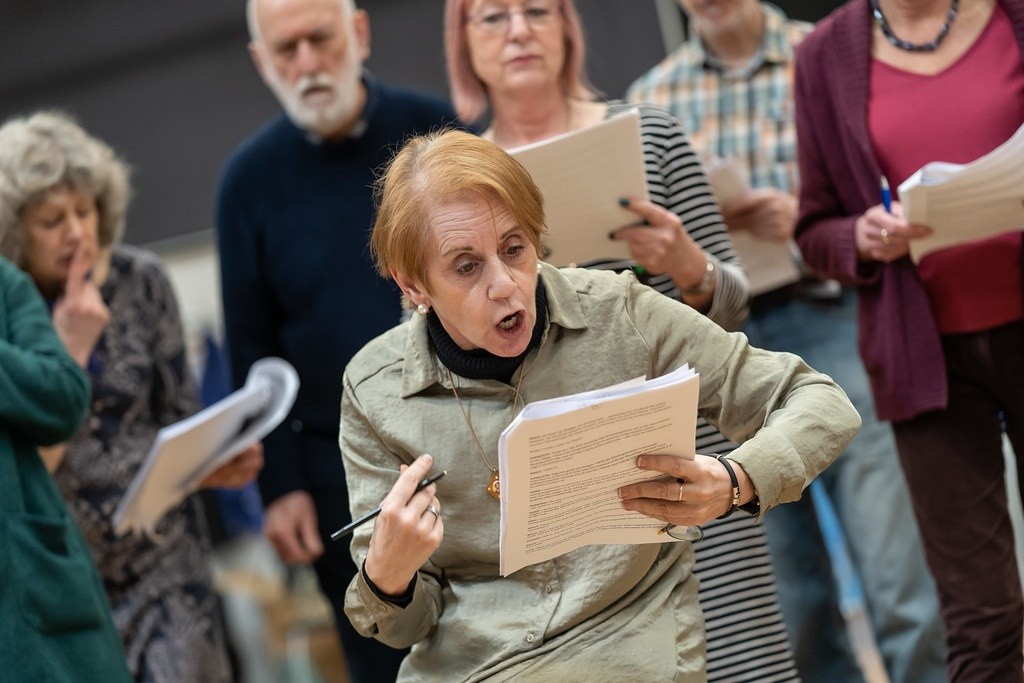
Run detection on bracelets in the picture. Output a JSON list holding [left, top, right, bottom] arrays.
[[706, 453, 740, 520], [676, 254, 714, 296]]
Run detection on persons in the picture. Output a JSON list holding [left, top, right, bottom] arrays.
[[793, 0, 1023, 683], [443, 0, 803, 683], [620, 0, 948, 682], [0, 110, 265, 683], [337, 130, 863, 683], [217, 0, 474, 683]]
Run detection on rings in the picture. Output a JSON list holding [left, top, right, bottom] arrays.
[[880, 229, 890, 245], [426, 505, 440, 520], [678, 483, 683, 502]]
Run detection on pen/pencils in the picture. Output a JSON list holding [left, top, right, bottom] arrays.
[[330, 470, 449, 541], [881, 175, 891, 213]]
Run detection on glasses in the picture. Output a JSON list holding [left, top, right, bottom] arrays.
[[468, 2, 558, 33], [658, 523, 703, 542]]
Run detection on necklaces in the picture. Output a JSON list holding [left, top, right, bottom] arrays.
[[446, 358, 527, 502], [873, 0, 958, 52]]
